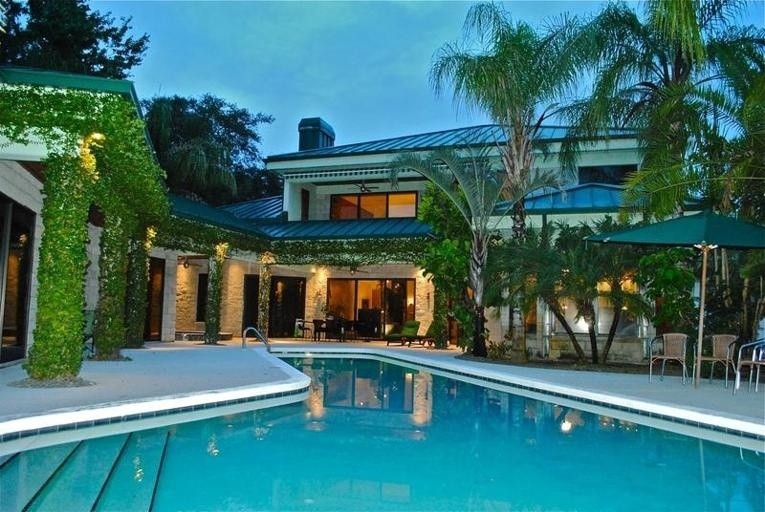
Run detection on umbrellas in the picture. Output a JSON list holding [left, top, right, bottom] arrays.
[[582, 208, 765, 390]]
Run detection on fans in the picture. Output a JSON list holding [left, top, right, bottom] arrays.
[[347, 180, 379, 194]]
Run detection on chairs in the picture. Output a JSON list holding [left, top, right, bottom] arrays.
[[692, 331, 742, 389], [732, 337, 764, 396], [647, 332, 689, 385], [299, 316, 441, 349]]
